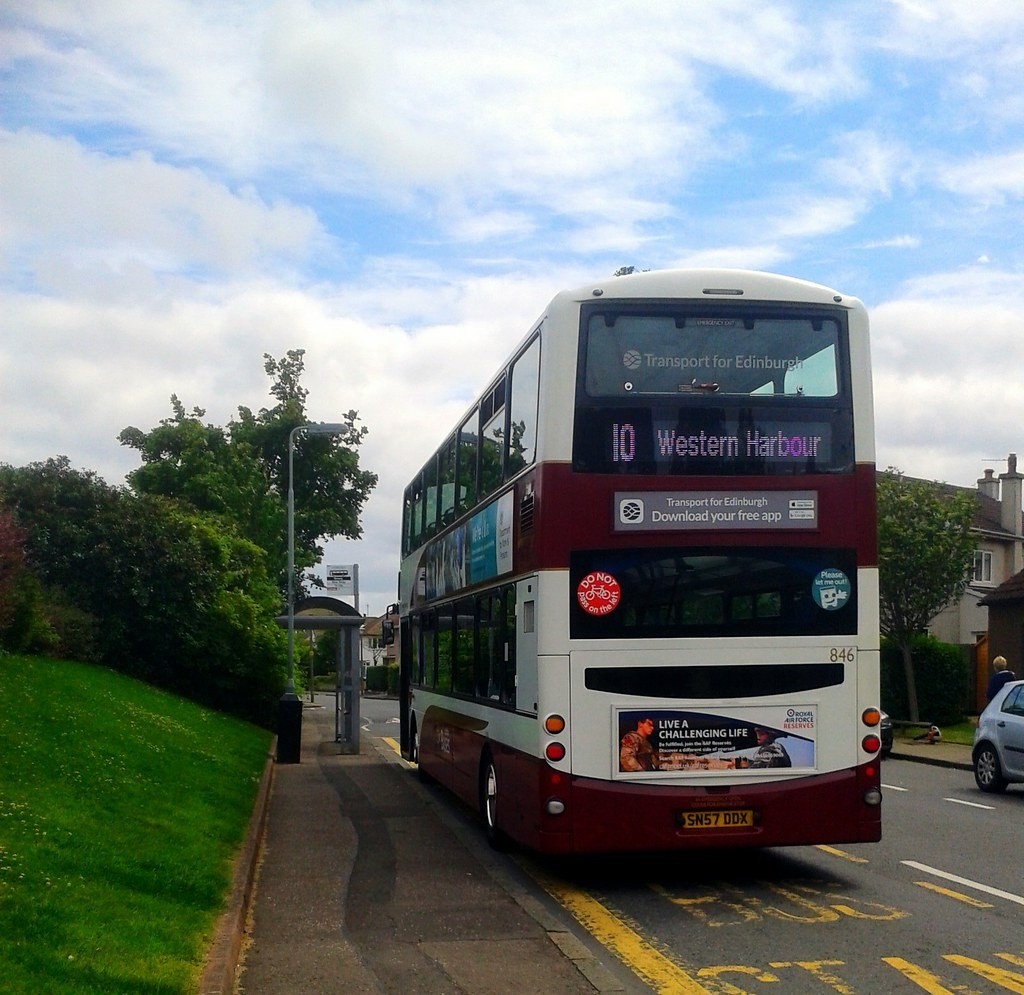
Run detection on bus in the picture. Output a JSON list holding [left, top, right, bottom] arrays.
[[381, 268, 884, 856]]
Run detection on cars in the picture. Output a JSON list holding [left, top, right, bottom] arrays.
[[972, 680, 1024, 794]]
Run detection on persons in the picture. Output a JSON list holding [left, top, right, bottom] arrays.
[[987, 656, 1017, 702], [620, 718, 672, 772], [747, 727, 792, 768]]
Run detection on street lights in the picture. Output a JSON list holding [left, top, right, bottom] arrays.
[[286, 424, 351, 695]]
